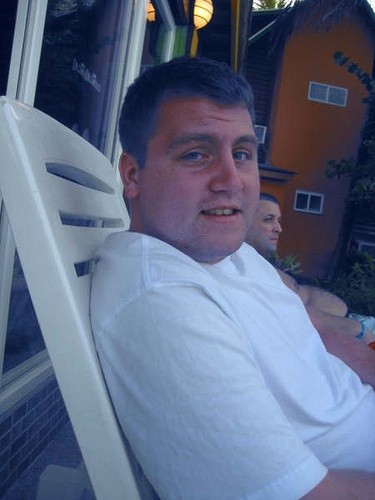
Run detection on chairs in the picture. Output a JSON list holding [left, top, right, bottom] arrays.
[[1, 96, 158, 500]]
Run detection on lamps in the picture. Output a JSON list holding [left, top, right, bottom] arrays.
[[147, 1, 155, 23], [193, 0, 214, 30]]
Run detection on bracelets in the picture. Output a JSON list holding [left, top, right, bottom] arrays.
[[355, 322, 366, 340]]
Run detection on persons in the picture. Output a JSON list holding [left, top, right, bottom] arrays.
[[89, 56, 375, 500], [243, 192, 375, 346]]
